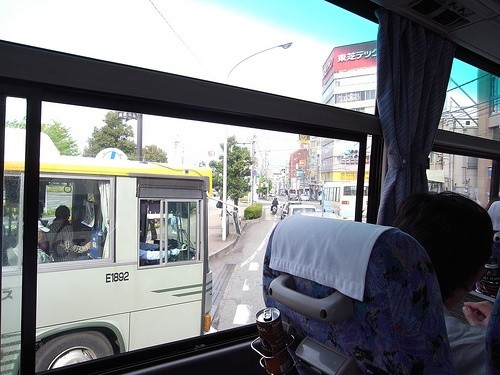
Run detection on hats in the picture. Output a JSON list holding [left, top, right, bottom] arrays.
[[38, 220, 50, 232]]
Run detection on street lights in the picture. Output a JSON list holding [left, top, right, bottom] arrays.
[[221, 40, 293, 241]]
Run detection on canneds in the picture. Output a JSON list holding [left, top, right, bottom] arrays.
[[255, 307, 291, 374], [480, 263, 499, 298]]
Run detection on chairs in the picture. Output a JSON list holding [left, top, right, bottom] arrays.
[[248, 213, 458, 375], [6, 246, 18, 267], [475, 199, 500, 298]]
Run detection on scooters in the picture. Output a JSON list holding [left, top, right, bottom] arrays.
[[272, 206, 277, 214]]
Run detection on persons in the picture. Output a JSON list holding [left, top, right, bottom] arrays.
[[393, 191, 496, 375], [271, 198, 278, 211], [36, 206, 92, 263], [139, 234, 181, 261]]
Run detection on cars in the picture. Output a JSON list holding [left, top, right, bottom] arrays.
[[280, 193, 319, 218]]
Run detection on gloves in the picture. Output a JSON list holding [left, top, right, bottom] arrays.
[[171, 248, 180, 255], [162, 242, 166, 249]]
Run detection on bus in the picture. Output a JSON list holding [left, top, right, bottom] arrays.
[[0, 158, 217, 375], [321, 181, 369, 220]]
